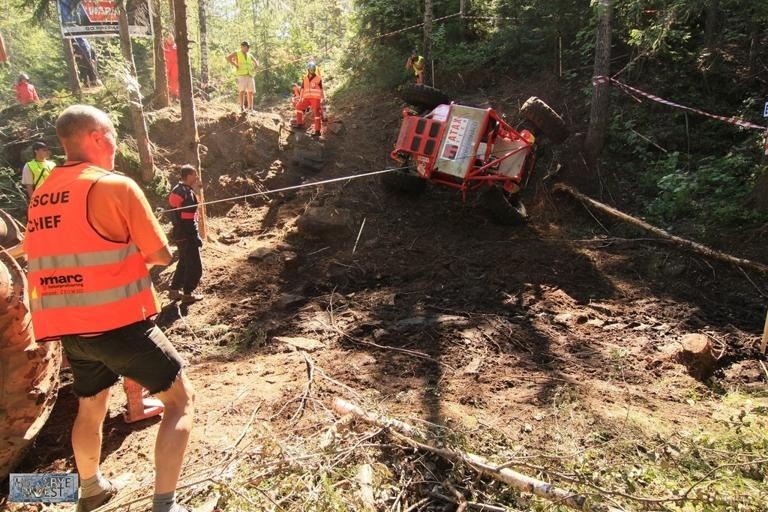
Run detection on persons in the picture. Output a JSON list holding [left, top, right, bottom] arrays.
[[225, 40, 259, 117], [166, 165, 206, 305], [23, 104, 196, 512], [408, 50, 427, 85], [21, 140, 58, 202], [14, 74, 40, 106], [293, 62, 326, 138]]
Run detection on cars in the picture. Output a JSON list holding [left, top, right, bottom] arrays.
[[381, 82, 573, 228]]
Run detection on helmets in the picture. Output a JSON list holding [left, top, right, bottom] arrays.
[[19, 74, 29, 80], [241, 41, 249, 46], [308, 63, 315, 68]]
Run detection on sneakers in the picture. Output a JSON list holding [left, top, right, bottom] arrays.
[[74, 472, 135, 512], [293, 124, 303, 129], [240, 108, 254, 116], [168, 290, 204, 302], [311, 131, 321, 136]]
